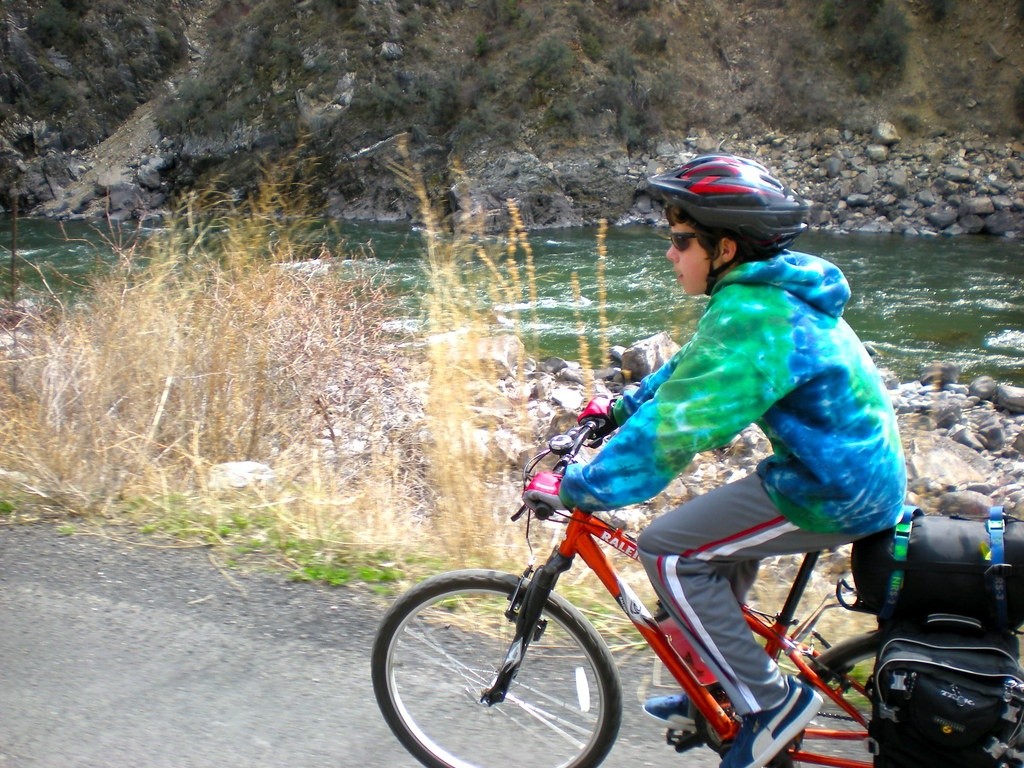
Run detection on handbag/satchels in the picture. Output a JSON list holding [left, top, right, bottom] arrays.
[[850, 504, 1024, 633], [864, 633, 1024, 768]]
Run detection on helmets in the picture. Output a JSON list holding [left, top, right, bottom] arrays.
[[637, 155, 810, 259]]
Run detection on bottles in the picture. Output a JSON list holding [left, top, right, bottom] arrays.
[[653, 599, 718, 684]]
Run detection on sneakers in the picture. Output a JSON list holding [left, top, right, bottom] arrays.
[[719, 673, 824, 767], [640, 692, 700, 734]]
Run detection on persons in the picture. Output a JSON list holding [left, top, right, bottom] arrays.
[[521, 154, 907, 768]]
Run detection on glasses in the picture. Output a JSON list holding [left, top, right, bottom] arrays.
[[669, 232, 705, 252]]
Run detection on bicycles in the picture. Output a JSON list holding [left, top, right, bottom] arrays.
[[370, 418, 1024, 768]]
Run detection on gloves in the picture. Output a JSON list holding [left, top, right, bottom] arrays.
[[522, 470, 569, 519], [577, 396, 618, 441]]
